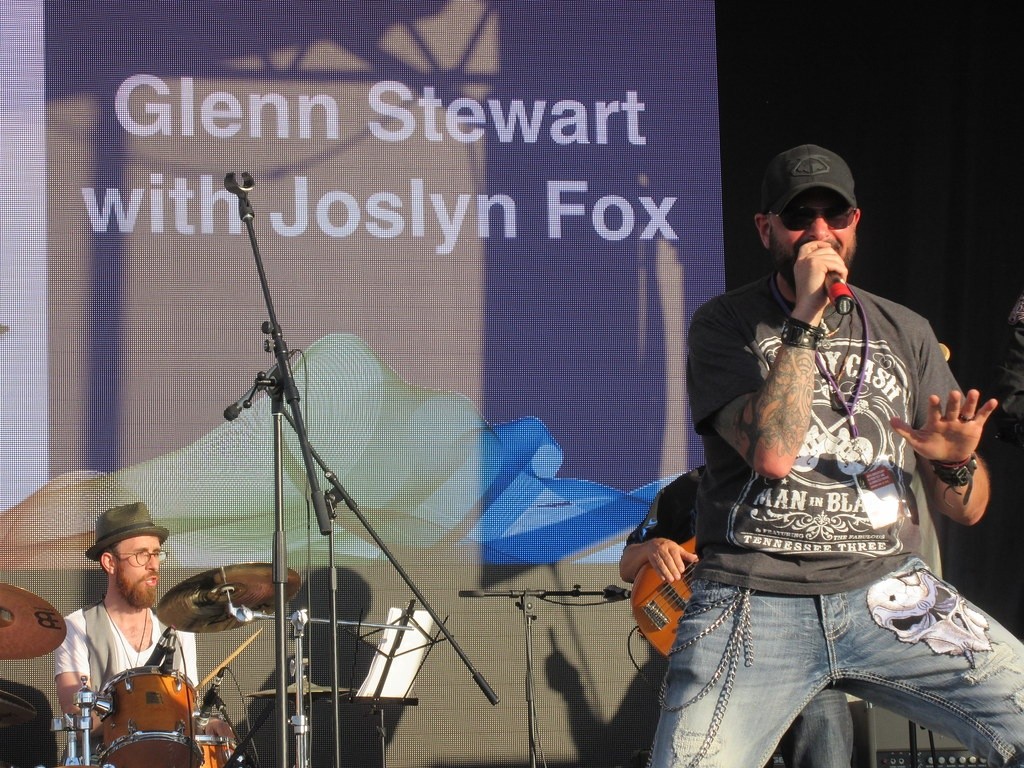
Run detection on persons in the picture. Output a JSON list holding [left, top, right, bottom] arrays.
[[618, 461, 855, 768], [648, 144, 1024, 768], [54, 501, 237, 768]]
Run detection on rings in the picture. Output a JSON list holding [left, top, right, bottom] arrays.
[[960, 413, 976, 422]]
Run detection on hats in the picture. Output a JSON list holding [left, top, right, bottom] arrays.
[[85, 502, 170, 561], [760, 144, 856, 213]]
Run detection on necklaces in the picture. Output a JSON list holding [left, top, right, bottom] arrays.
[[774, 270, 853, 413], [102, 595, 147, 668]]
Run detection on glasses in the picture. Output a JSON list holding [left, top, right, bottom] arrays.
[[109, 550, 168, 566], [778, 205, 856, 230]]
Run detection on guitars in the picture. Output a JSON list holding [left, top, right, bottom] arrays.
[[627, 341, 953, 661]]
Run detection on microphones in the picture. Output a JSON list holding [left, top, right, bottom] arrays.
[[146, 626, 175, 666], [604, 584, 632, 599], [224, 349, 296, 421], [823, 269, 855, 315], [196, 669, 224, 727]]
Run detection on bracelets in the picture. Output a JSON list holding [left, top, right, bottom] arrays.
[[930, 450, 978, 506], [202, 727, 206, 734], [780, 316, 824, 351]]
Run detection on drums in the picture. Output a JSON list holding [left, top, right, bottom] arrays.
[[98, 664, 203, 768], [194, 734, 240, 768]]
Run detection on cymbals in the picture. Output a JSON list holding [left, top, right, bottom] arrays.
[[244, 679, 358, 702], [155, 561, 303, 634], [0, 581, 68, 661], [0, 688, 39, 726]]
